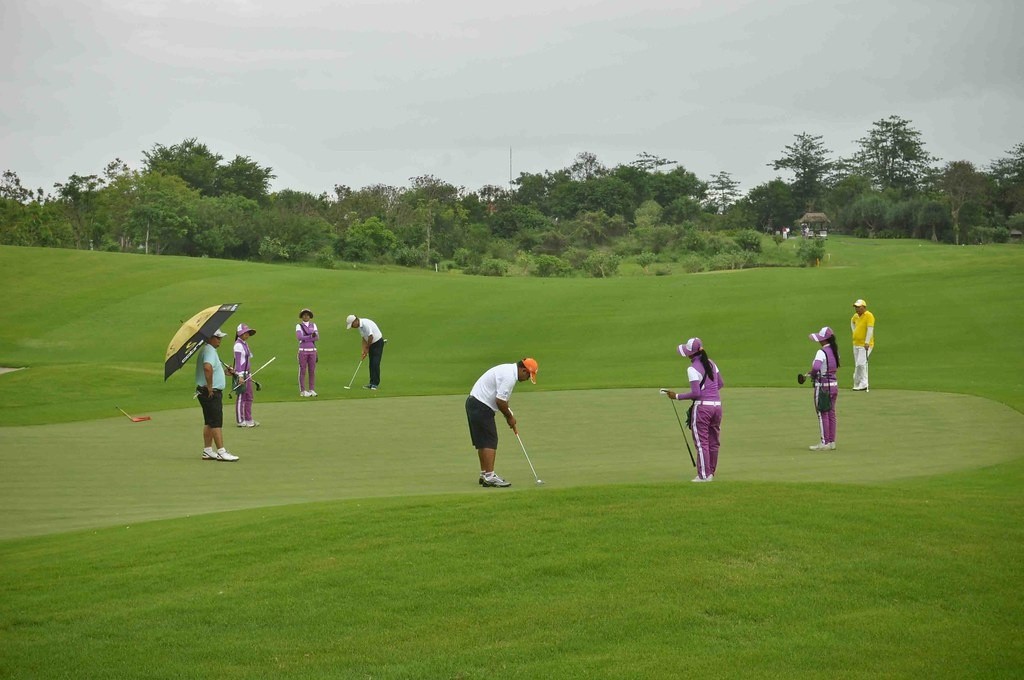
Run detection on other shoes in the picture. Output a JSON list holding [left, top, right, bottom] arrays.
[[852, 385, 867, 391], [691, 474, 713, 483], [363, 385, 373, 390], [371, 385, 379, 390]]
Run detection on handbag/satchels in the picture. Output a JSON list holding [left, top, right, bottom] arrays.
[[817, 390, 832, 411], [231, 373, 246, 396], [686, 405, 694, 430]]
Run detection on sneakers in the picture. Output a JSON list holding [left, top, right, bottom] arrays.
[[201, 450, 218, 459], [483, 477, 511, 487], [237, 419, 260, 427], [478, 474, 485, 484], [809, 437, 836, 451], [299, 390, 312, 397], [217, 451, 239, 461], [309, 389, 318, 397]]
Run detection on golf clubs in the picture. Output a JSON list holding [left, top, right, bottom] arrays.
[[228, 356, 276, 399], [659, 388, 697, 468], [221, 360, 262, 391], [866, 350, 870, 392], [512, 427, 542, 483], [343, 355, 365, 390]]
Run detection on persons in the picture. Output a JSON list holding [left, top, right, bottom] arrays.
[[345, 315, 384, 390], [232, 322, 260, 428], [194, 328, 240, 461], [851, 299, 875, 391], [667, 337, 723, 483], [465, 358, 539, 488], [782, 225, 792, 233], [803, 225, 809, 246], [822, 224, 828, 231], [296, 308, 320, 397], [806, 326, 841, 450]]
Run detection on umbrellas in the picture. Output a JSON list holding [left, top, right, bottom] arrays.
[[164, 303, 239, 382]]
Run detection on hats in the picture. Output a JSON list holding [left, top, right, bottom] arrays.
[[347, 315, 356, 330], [853, 299, 866, 307], [213, 329, 227, 338], [236, 324, 256, 336], [809, 326, 834, 342], [676, 336, 703, 356], [521, 357, 539, 384], [299, 310, 313, 318]]
